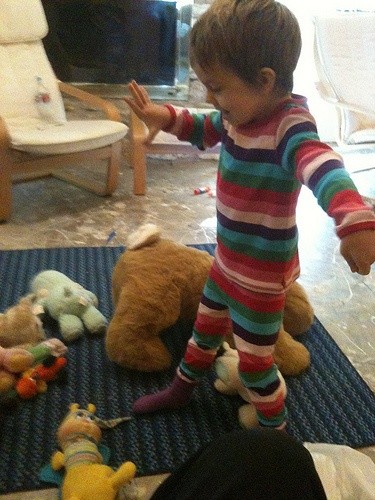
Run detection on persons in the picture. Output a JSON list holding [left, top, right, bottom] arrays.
[[125, 0, 375, 431], [148, 435, 374, 500]]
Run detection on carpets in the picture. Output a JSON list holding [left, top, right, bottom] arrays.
[[0, 243, 375, 495]]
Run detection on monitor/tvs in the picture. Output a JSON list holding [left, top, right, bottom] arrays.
[[39, 0, 193, 101]]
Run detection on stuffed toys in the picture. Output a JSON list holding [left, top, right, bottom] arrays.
[[52, 402, 136, 500], [0, 289, 68, 399], [32, 269, 108, 342], [212, 341, 287, 428], [106, 225, 314, 377]]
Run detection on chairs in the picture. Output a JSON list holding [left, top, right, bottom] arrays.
[[0, 0, 130, 225]]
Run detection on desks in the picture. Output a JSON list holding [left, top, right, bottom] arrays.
[[130, 108, 221, 197]]
